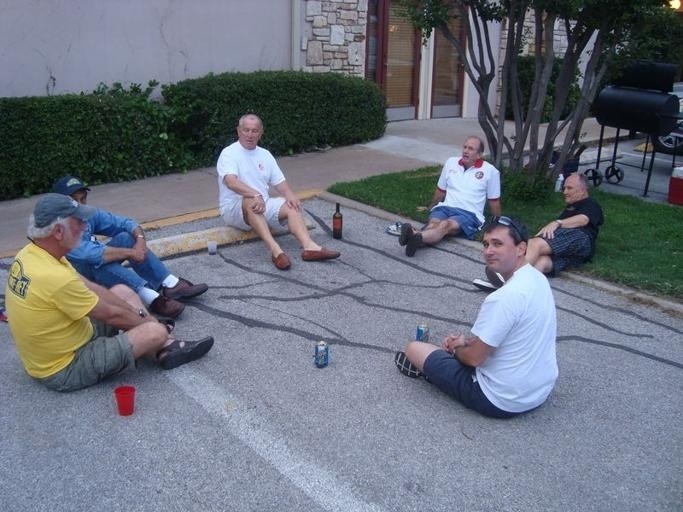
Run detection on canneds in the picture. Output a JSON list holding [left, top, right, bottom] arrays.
[[315, 340, 329, 368], [416, 322, 429, 342]]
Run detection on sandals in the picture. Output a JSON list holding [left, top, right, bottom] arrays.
[[156, 337, 214, 370], [112, 317, 175, 335]]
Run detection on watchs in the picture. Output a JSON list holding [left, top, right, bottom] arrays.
[[556, 220, 563, 228], [139, 309, 146, 318]]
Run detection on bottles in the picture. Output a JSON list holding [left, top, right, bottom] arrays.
[[208, 241, 217, 255], [333, 202, 343, 239]]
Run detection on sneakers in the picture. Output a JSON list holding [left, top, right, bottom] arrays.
[[485, 266, 505, 287], [399, 223, 413, 246], [395, 352, 431, 382], [406, 233, 422, 256], [473, 278, 498, 292]]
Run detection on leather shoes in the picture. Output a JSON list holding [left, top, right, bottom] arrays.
[[149, 295, 185, 317], [303, 247, 340, 260], [160, 277, 208, 299], [272, 253, 291, 269]]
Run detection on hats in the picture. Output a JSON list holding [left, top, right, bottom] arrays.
[[33, 193, 97, 228], [53, 175, 91, 195]]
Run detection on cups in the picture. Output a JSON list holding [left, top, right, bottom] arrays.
[[115, 386, 135, 416]]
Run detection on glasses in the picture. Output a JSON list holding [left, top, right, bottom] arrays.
[[481, 216, 527, 242]]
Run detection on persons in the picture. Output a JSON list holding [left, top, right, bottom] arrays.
[[398, 135, 501, 257], [5, 193, 214, 392], [51, 174, 208, 319], [216, 113, 340, 270], [472, 173, 604, 291], [394, 215, 559, 420]]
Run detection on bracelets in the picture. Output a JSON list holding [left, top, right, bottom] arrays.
[[255, 194, 263, 197], [136, 234, 146, 238]]
